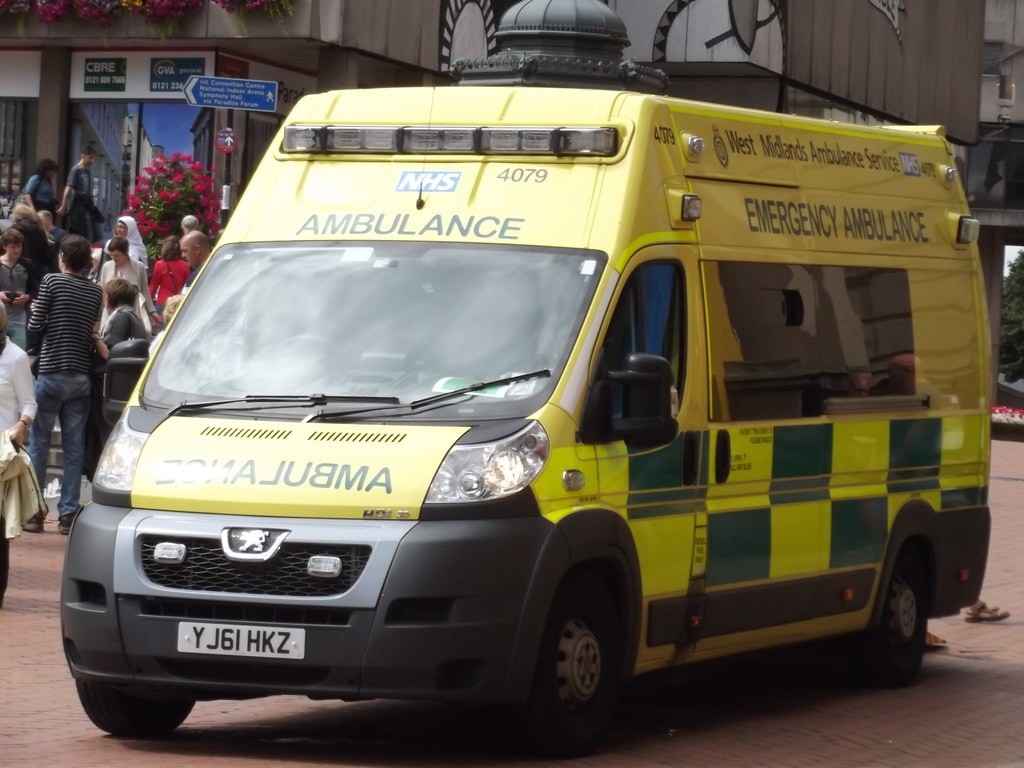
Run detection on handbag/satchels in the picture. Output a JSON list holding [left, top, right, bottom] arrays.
[[90, 349, 113, 374]]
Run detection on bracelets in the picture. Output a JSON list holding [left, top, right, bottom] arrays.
[[95, 338, 102, 341], [19, 419, 27, 426]]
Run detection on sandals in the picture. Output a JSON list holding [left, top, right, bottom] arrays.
[[964, 601, 1011, 623]]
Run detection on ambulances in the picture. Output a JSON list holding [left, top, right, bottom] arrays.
[[59, 84, 997, 758]]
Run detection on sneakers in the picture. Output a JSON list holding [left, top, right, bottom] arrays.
[[58, 517, 73, 534], [23, 512, 44, 533]]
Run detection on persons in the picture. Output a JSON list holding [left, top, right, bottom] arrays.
[[925, 600, 1011, 646], [850, 355, 943, 476], [0, 145, 211, 608]]
